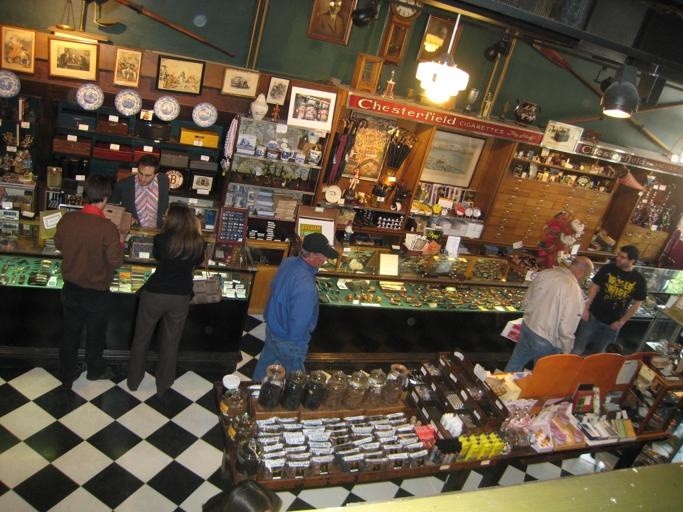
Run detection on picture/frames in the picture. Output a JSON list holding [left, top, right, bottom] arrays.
[[540, 119, 584, 156], [47, 35, 98, 84], [419, 130, 487, 188], [112, 46, 143, 89], [219, 66, 337, 131], [306, 0, 359, 48], [0, 25, 34, 76], [155, 54, 206, 98]]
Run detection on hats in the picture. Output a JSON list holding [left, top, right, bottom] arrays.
[[301, 233, 340, 260]]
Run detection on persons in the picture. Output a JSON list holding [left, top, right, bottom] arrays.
[[127, 204, 205, 397], [53, 172, 123, 380], [571, 245, 647, 356], [58, 51, 89, 70], [293, 98, 329, 122], [251, 232, 338, 381], [505, 256, 594, 371], [315, 1, 345, 39], [109, 155, 169, 229], [117, 63, 137, 81], [8, 36, 30, 65], [422, 25, 448, 61]]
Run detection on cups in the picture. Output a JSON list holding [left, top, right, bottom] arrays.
[[255, 140, 323, 167]]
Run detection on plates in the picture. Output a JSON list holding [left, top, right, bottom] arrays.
[[325, 184, 342, 203], [0, 70, 22, 99], [76, 83, 105, 110], [114, 89, 142, 118], [153, 95, 181, 123], [191, 103, 219, 128]]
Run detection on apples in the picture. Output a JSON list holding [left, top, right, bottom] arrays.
[[433, 204, 441, 213]]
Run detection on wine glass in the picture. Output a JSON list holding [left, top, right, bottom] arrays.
[[461, 86, 480, 116]]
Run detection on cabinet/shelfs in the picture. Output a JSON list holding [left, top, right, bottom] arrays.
[[244, 242, 291, 316], [296, 237, 529, 353], [630, 351, 682, 467], [577, 263, 682, 352], [1, 213, 257, 373], [41, 97, 221, 213], [224, 130, 328, 241], [476, 138, 616, 251], [0, 88, 39, 210], [602, 178, 682, 263], [403, 126, 517, 246], [314, 92, 436, 248]]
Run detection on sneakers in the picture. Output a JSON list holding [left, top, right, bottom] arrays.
[[155, 368, 169, 398], [60, 358, 113, 382], [127, 368, 145, 391]]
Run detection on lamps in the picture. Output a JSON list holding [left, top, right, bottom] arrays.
[[599, 61, 641, 121], [414, 12, 469, 105], [424, 22, 448, 52]]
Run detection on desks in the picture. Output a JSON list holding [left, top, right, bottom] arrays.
[[479, 371, 672, 486], [213, 381, 498, 491]]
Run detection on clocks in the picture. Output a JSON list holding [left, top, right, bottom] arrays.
[[378, 1, 424, 67]]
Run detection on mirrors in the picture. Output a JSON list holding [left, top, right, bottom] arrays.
[[417, 12, 464, 64]]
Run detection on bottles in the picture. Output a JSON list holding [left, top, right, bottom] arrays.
[[352, 209, 405, 231], [253, 359, 408, 412], [249, 92, 268, 121], [216, 371, 265, 476], [343, 218, 353, 241], [428, 431, 507, 466], [371, 182, 386, 210], [343, 177, 358, 206]]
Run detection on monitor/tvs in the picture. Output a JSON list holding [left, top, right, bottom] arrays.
[[298, 216, 336, 247]]
